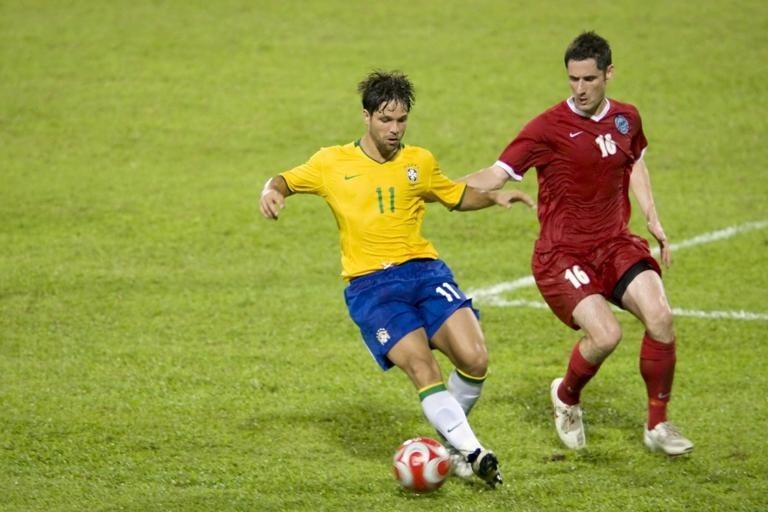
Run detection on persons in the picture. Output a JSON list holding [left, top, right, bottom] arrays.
[[258, 64, 540, 490], [451, 33, 701, 457]]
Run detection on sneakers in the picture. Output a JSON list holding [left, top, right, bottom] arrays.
[[437, 439, 504, 494], [643, 420, 695, 458], [550, 375, 586, 450]]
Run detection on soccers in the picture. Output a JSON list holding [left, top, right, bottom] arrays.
[[394, 437, 451, 493]]
